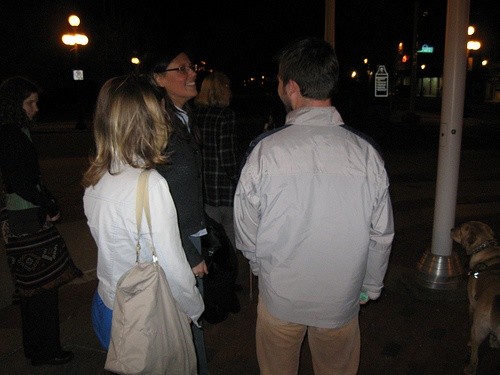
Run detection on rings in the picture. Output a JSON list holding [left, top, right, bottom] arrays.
[[195, 274, 199, 277]]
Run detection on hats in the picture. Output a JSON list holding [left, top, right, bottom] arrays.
[[136, 44, 181, 78]]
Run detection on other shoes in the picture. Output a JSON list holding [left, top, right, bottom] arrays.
[[23, 350, 73, 366]]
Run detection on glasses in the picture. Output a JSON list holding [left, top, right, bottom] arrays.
[[154, 64, 198, 73]]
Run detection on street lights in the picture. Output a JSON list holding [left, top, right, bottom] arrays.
[[61, 14, 91, 130]]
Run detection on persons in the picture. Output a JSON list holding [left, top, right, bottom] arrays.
[[136, 42, 207, 375], [0, 75, 75, 366], [192, 71, 248, 314], [232, 38, 395, 375], [80, 77, 205, 353]]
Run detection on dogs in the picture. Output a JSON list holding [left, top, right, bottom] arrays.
[[450, 220, 500, 375]]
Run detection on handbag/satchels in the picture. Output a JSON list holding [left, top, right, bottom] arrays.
[[104, 168, 197, 375]]
